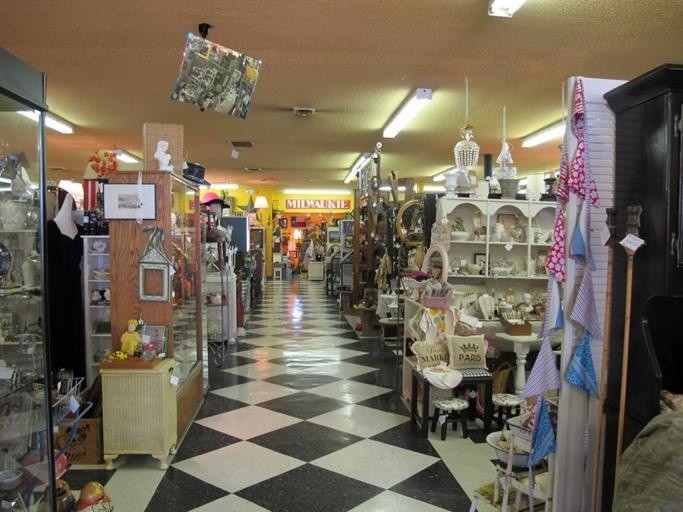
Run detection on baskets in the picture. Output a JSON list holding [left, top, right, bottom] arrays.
[[500, 317, 531, 336], [51, 376, 84, 426]]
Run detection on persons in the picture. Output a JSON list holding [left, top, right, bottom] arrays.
[[43, 190, 85, 383]]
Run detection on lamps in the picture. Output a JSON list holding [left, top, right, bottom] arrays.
[[253, 195, 269, 209], [283, 188, 352, 196], [18, 107, 239, 194], [342, 87, 567, 193], [488, 1, 528, 19]]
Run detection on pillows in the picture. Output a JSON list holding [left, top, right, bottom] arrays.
[[445, 332, 488, 370], [409, 338, 450, 372]]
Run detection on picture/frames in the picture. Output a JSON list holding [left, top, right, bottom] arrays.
[[279, 218, 287, 228], [289, 251, 297, 258]]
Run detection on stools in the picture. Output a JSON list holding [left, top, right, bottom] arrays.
[[493, 462, 548, 508], [405, 362, 496, 443], [490, 391, 524, 435], [273, 253, 283, 281], [430, 396, 473, 444]]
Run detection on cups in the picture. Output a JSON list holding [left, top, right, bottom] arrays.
[[3, 199, 29, 231]]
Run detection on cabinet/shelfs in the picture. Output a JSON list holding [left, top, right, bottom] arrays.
[[325, 193, 563, 340], [0, 45, 266, 511], [307, 260, 324, 281]]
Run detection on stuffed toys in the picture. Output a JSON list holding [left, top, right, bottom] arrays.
[[119, 318, 141, 357], [107, 352, 128, 361]]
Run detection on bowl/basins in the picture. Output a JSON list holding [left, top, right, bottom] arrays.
[[486, 430, 529, 467], [451, 230, 470, 241]]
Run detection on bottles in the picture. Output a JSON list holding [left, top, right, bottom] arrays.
[[507, 288, 533, 306], [83, 208, 97, 236], [491, 222, 505, 242], [21, 261, 34, 287]]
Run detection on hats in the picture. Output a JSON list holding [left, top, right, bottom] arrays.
[[184, 162, 211, 186], [201, 198, 230, 208]]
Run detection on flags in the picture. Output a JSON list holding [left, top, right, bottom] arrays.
[[82, 178, 108, 217]]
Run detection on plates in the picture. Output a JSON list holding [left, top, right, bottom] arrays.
[[0, 470, 24, 484]]
